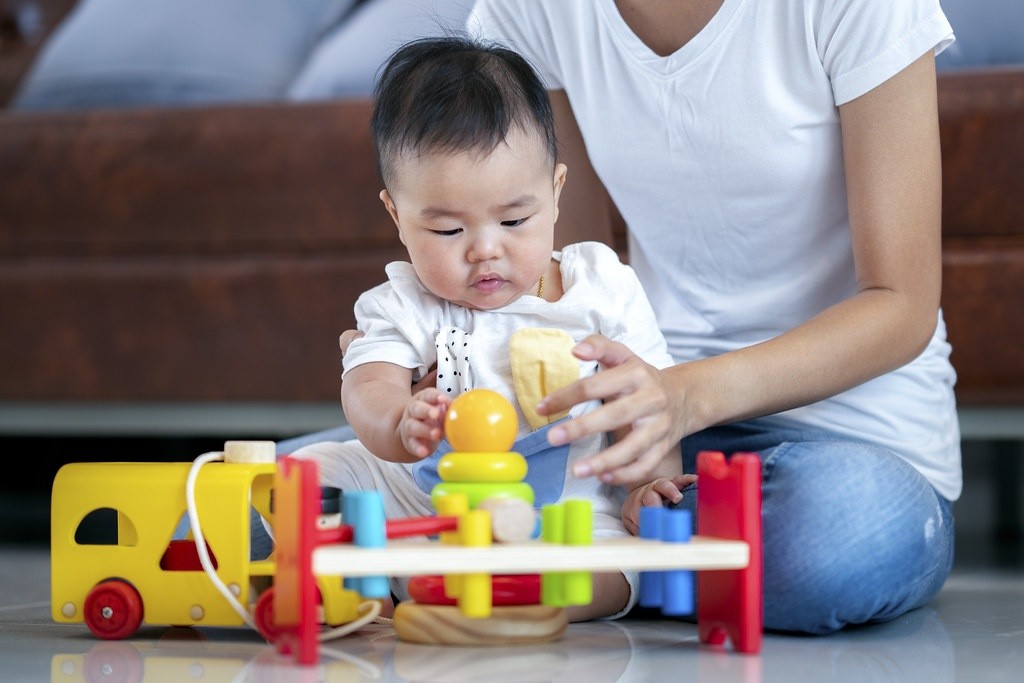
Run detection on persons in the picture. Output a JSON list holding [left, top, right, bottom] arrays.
[[171, 0, 964, 640], [260, 37, 700, 646]]
